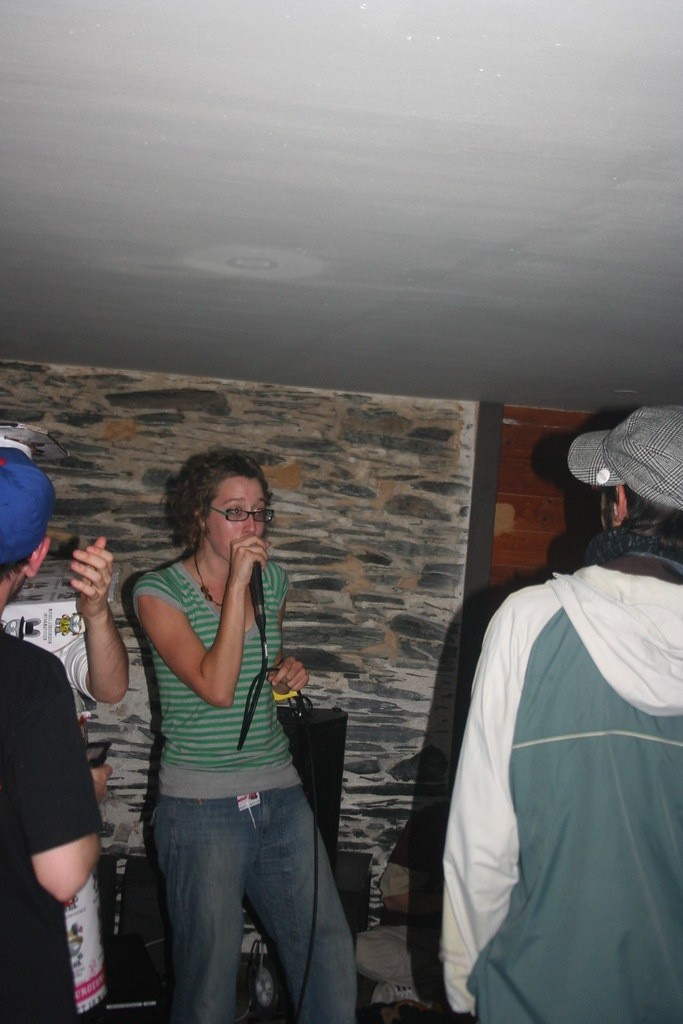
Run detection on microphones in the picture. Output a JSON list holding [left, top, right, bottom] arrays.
[[249, 561, 267, 631]]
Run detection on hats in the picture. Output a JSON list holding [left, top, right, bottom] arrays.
[[567, 404, 683, 511], [0, 446, 55, 563]]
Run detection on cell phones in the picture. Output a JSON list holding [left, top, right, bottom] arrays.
[[84, 741, 111, 770]]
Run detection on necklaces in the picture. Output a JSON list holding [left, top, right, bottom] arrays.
[[195, 552, 223, 608]]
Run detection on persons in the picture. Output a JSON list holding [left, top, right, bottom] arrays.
[[0, 421, 130, 1024], [440, 405, 683, 1024], [132, 448, 357, 1024]]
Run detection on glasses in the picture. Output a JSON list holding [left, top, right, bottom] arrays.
[[207, 506, 274, 522]]
